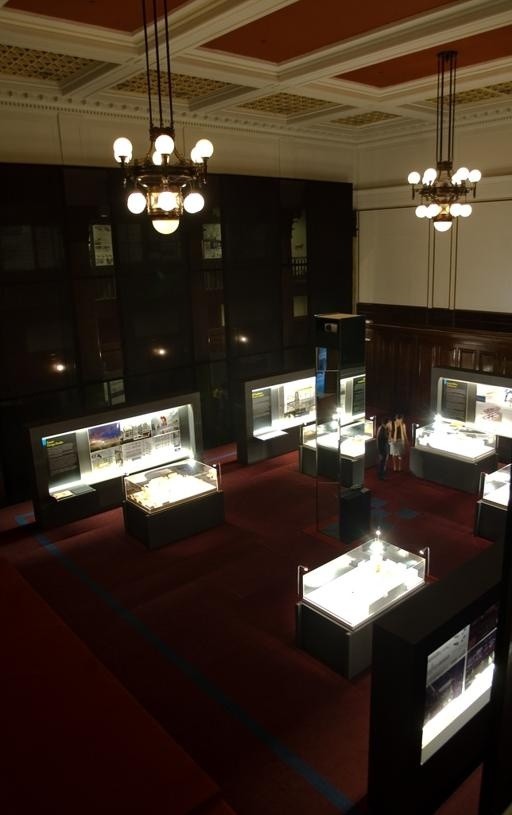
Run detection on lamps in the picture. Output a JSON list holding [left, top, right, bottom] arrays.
[[405, 49, 481, 234], [114, 1, 215, 236]]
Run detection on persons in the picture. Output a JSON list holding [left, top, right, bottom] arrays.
[[374, 415, 393, 482], [387, 414, 411, 473]]
